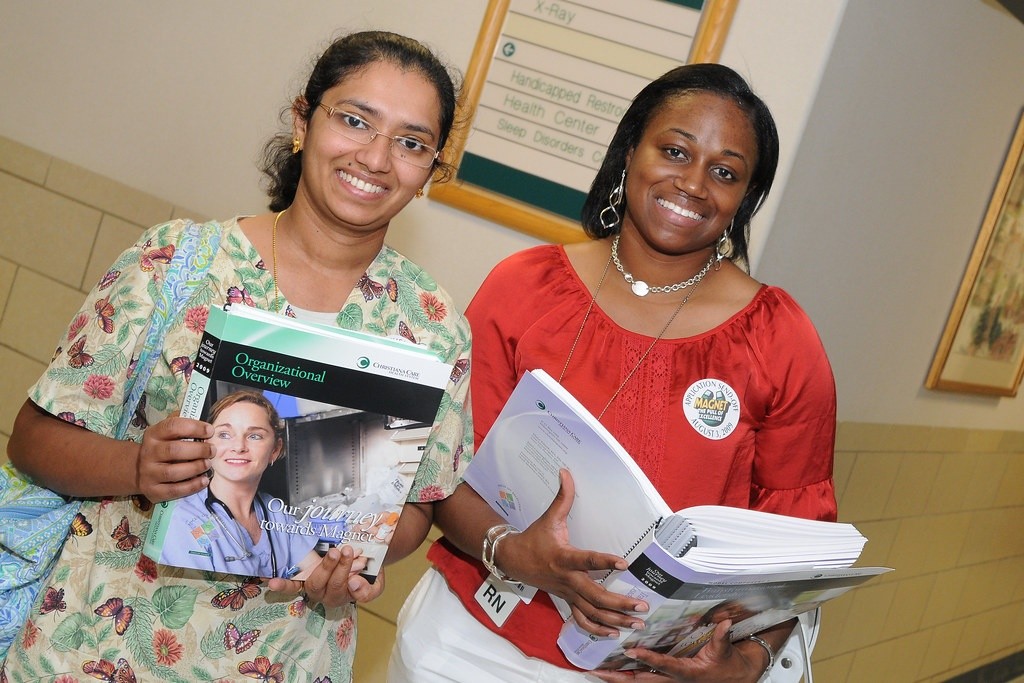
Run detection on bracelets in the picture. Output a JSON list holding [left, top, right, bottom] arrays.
[[482, 525, 521, 584], [749, 635, 775, 676]]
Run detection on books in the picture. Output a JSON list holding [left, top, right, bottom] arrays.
[[142, 305, 454, 583], [463, 371, 895, 671]]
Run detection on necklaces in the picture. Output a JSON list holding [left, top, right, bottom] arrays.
[[273, 209, 287, 313], [558, 235, 717, 422]]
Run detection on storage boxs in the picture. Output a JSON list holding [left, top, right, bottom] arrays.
[[308, 515, 348, 543]]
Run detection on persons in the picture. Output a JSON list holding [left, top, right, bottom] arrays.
[[159, 392, 366, 581], [0, 32, 474, 683], [382, 62, 836, 683], [662, 593, 829, 658]]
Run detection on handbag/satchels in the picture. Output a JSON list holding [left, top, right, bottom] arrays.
[[0, 220, 224, 661]]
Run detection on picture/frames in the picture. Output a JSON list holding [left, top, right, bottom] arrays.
[[923, 115, 1024, 399]]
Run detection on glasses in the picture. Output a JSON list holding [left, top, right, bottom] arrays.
[[314, 98, 439, 168]]
